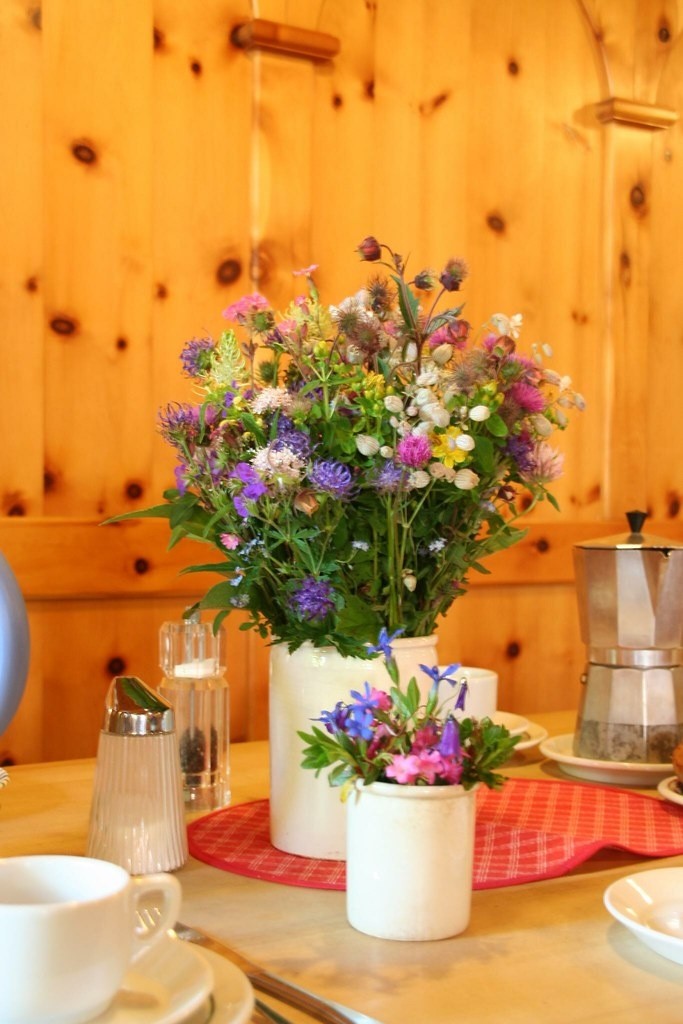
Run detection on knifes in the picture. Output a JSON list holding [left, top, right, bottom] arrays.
[[172, 920, 385, 1024]]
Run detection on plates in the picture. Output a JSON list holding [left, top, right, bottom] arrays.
[[602, 866, 683, 967], [169, 942, 255, 1024], [90, 938, 217, 1024], [513, 722, 548, 750], [538, 731, 676, 785], [494, 710, 530, 737], [657, 775, 683, 806]]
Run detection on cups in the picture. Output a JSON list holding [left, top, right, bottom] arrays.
[[0, 854, 183, 1024], [437, 665, 499, 722]]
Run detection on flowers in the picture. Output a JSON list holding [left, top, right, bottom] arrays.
[[92, 235, 588, 661], [292, 624, 521, 802]]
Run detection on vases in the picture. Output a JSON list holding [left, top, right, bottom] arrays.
[[344, 776, 479, 941], [267, 629, 441, 864]]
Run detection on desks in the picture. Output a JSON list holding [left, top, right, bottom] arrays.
[[0, 705, 683, 1024]]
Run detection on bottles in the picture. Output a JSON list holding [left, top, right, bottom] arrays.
[[156, 619, 232, 817], [84, 705, 190, 876]]
